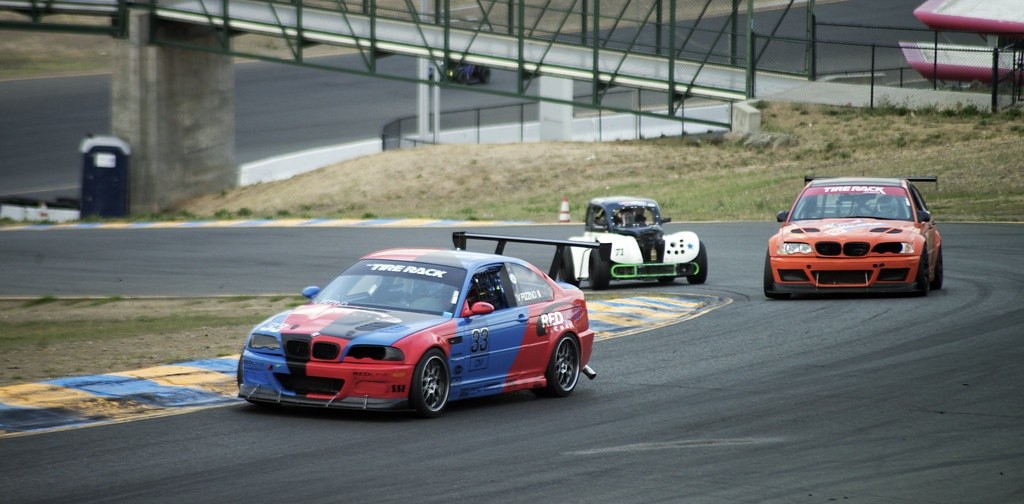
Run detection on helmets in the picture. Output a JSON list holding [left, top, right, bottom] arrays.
[[875, 195, 898, 218]]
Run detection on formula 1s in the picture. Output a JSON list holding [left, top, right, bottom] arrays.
[[761, 176, 944, 302], [237, 230, 615, 416]]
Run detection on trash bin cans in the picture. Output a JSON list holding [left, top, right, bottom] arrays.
[[78, 132, 131, 219]]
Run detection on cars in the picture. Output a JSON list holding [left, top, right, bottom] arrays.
[[557, 198, 709, 286]]
